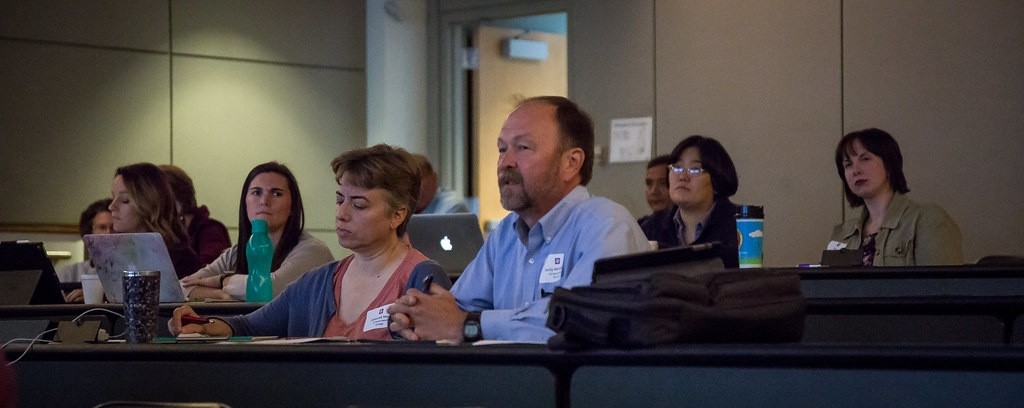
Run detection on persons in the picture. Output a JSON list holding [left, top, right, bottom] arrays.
[[388, 96, 650, 347], [639, 134, 762, 269], [176, 162, 334, 302], [168, 143, 453, 342], [409, 154, 467, 216], [156, 165, 233, 268], [634, 156, 677, 226], [55, 199, 121, 284], [828, 127, 963, 268], [67, 163, 203, 303]]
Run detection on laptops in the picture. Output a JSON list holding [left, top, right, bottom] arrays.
[[407, 213, 484, 277], [82, 233, 222, 303], [0, 240, 83, 304]]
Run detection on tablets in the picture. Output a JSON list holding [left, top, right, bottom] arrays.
[[591, 240, 721, 283]]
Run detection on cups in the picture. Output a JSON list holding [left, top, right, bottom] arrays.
[[122, 270, 161, 343], [648, 241, 658, 252], [733, 205, 765, 268], [81, 275, 104, 304]]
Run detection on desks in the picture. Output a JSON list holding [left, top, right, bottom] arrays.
[[0, 263, 1024, 408]]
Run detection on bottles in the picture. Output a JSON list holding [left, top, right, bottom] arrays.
[[245, 218, 274, 302]]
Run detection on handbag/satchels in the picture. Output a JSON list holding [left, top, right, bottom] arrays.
[[547, 241, 805, 349]]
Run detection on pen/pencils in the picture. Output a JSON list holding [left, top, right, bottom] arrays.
[[181, 315, 215, 325], [540, 288, 546, 298], [420, 274, 434, 294]]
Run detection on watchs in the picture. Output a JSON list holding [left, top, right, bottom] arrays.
[[463, 311, 484, 343]]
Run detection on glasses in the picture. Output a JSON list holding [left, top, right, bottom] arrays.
[[665, 163, 704, 176]]
